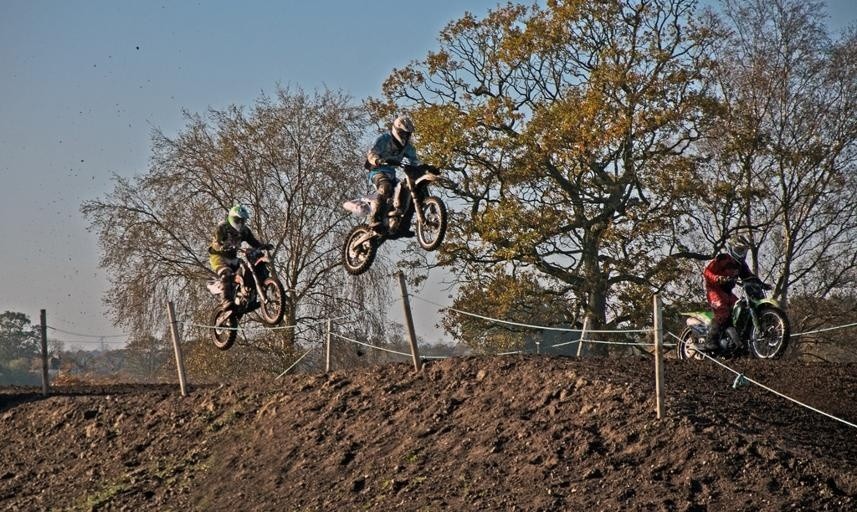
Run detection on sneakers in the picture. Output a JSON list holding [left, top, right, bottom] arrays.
[[703, 341, 719, 349], [371, 217, 382, 227], [223, 298, 233, 309]]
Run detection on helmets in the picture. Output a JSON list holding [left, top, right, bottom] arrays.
[[727, 235, 749, 265], [228, 203, 249, 233], [392, 117, 413, 146]]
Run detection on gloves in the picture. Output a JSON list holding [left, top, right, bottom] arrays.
[[719, 276, 727, 285], [386, 157, 400, 167], [261, 244, 273, 250]]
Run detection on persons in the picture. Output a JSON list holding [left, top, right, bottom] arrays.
[[364, 115, 421, 237], [704, 238, 773, 351], [208, 205, 274, 311]]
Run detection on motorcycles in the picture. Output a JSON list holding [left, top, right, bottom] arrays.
[[342, 163, 448, 274], [210, 241, 285, 347], [676, 278, 791, 358]]
